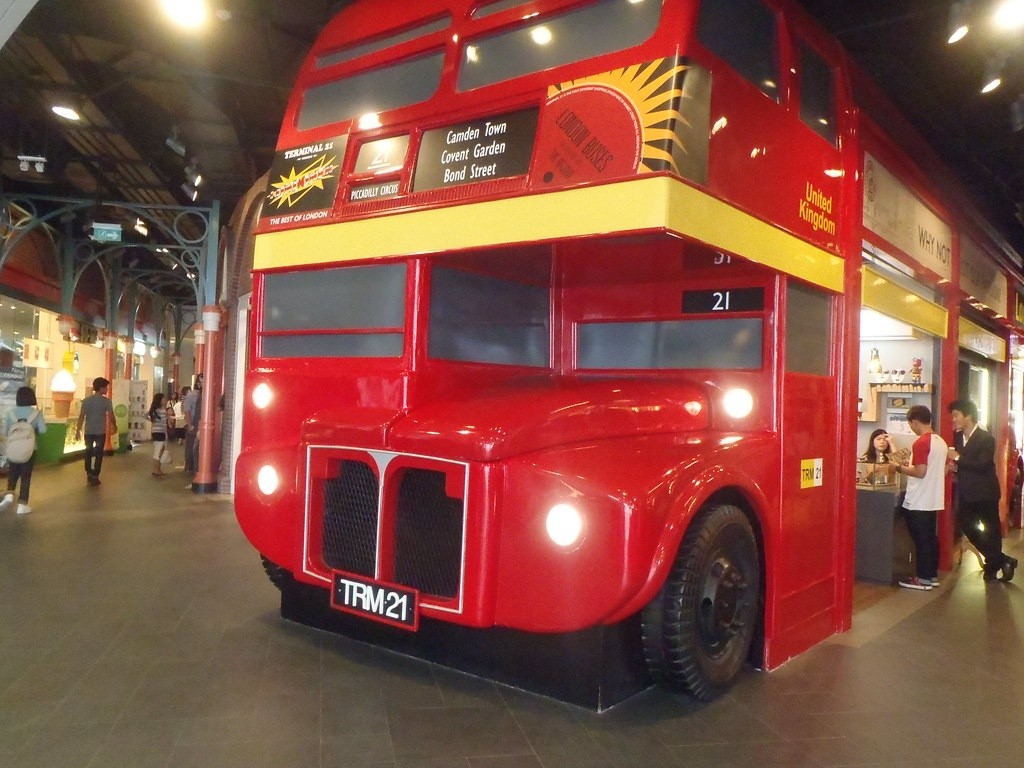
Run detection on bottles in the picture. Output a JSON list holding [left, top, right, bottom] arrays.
[[858, 398, 863, 421], [856, 471, 887, 487]]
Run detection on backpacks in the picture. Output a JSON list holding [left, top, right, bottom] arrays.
[[5, 409, 39, 464]]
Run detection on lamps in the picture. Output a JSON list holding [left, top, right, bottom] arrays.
[[969, 62, 1002, 95], [183, 163, 201, 187], [946, 0, 972, 44], [51, 91, 82, 120], [165, 125, 186, 157], [1009, 101, 1024, 132], [181, 183, 198, 201], [159, 255, 177, 270]]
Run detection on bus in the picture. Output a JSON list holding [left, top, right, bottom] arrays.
[[234, 0, 1024, 716]]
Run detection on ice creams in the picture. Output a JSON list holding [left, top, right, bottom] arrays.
[[51, 369, 76, 418]]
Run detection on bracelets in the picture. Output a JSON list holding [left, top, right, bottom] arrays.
[[895, 465, 901, 473]]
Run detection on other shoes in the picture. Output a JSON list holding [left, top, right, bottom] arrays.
[[88, 475, 101, 485], [999, 558, 1016, 582], [152, 473, 166, 476], [984, 567, 997, 579]]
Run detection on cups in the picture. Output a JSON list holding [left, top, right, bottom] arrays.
[[949, 447, 955, 451]]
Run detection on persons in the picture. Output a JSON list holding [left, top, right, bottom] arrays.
[[948, 400, 1018, 583], [861, 429, 897, 463], [0, 387, 47, 514], [142, 392, 169, 476], [75, 376, 117, 485], [166, 374, 225, 490], [887, 405, 948, 590]]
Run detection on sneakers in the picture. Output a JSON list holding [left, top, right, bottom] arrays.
[[17, 504, 31, 514], [931, 581, 940, 586], [898, 577, 931, 590], [0, 495, 13, 511]]
